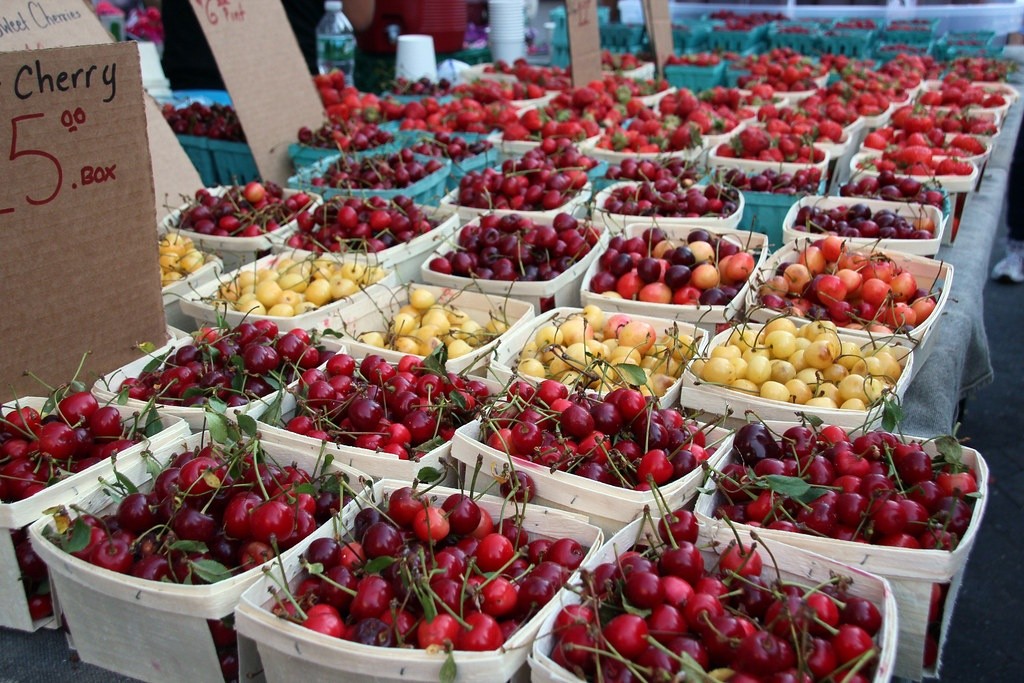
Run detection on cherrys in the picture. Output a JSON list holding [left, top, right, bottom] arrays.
[[0, 99, 982, 683]]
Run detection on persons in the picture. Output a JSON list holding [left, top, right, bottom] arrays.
[[990, 110, 1024, 283], [355, 0, 468, 56], [160, 0, 375, 90]]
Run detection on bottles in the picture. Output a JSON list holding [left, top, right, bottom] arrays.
[[315, 0, 356, 92]]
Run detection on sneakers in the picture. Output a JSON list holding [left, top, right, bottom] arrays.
[[993, 246, 1024, 283]]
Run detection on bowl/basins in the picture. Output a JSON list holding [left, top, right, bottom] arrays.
[[400, 0, 467, 53]]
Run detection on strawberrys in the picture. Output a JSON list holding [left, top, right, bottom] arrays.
[[312, 11, 1010, 239]]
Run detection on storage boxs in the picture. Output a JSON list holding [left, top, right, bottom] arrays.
[[0, 6, 1024, 683]]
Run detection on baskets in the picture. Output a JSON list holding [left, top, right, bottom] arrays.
[[172, 7, 1005, 251]]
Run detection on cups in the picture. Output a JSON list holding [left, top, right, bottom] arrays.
[[394, 34, 437, 86], [486, 0, 525, 64]]
